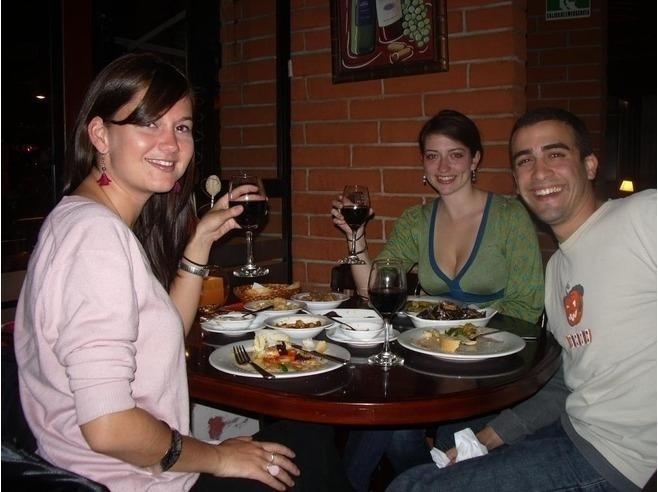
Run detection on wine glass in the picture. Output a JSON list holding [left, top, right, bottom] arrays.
[[334, 182, 371, 264], [226, 175, 272, 279], [371, 369, 397, 401], [365, 258, 408, 370]]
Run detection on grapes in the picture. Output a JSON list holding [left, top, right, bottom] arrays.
[[401, 0, 431, 47]]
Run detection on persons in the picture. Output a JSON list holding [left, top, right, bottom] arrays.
[[377, 105, 656, 491], [11, 46, 355, 492], [328, 106, 547, 492]]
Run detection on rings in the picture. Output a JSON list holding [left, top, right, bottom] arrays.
[[269, 451, 279, 463], [266, 463, 281, 476]]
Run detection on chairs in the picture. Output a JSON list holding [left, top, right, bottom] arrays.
[[1, 321, 109, 492], [169, 264, 229, 307], [331, 264, 417, 292]]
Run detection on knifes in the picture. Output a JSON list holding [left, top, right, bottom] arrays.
[[287, 339, 353, 368]]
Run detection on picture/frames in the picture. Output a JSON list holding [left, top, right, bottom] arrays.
[[329, 0, 447, 84]]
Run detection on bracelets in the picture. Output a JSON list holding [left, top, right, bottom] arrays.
[[346, 232, 369, 257], [140, 416, 184, 477], [176, 253, 211, 280]]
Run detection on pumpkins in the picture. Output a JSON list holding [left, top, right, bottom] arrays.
[[563, 285, 584, 327]]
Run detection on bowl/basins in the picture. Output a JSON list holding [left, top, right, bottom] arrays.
[[244, 299, 305, 314], [263, 314, 333, 337], [340, 321, 384, 338], [292, 292, 351, 309], [404, 306, 497, 329], [232, 284, 300, 298], [214, 312, 255, 330]]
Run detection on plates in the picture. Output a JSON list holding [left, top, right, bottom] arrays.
[[271, 369, 351, 396], [326, 328, 400, 349], [201, 316, 266, 337], [396, 326, 527, 364], [200, 333, 235, 347], [205, 336, 352, 380], [316, 305, 386, 324], [398, 355, 527, 381]]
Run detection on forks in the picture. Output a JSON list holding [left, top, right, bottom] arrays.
[[232, 343, 275, 381]]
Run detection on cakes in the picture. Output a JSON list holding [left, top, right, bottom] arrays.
[[253, 329, 289, 358]]
